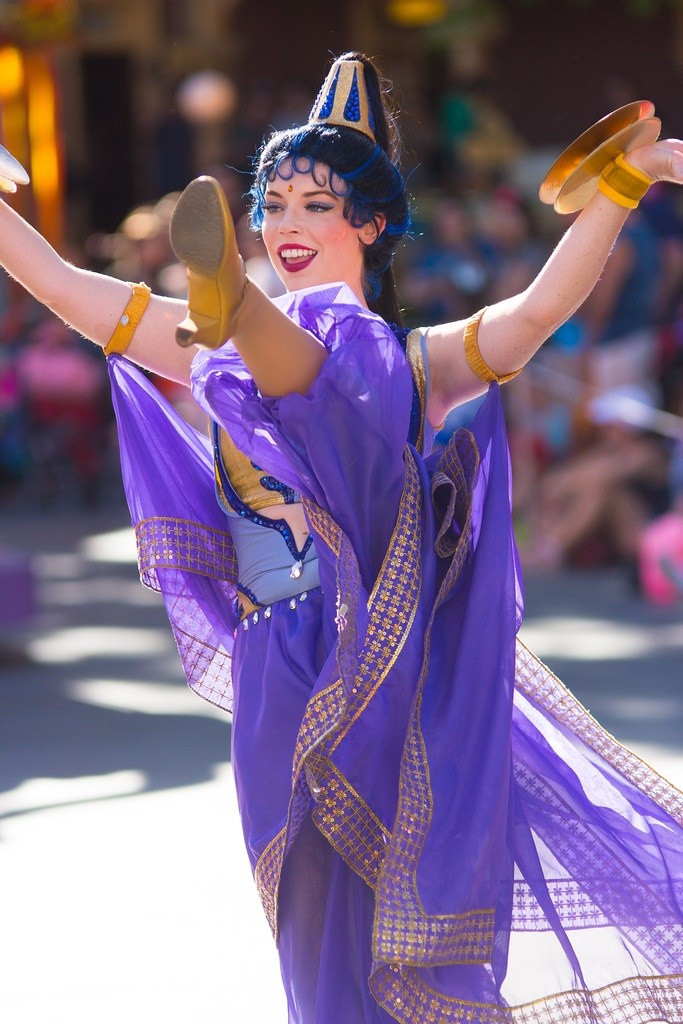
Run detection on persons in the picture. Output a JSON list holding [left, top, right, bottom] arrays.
[[0, 189, 288, 506], [407, 148, 682, 604], [0, 51, 683, 1024]]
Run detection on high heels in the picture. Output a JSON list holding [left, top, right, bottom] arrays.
[[170, 175, 245, 352]]
